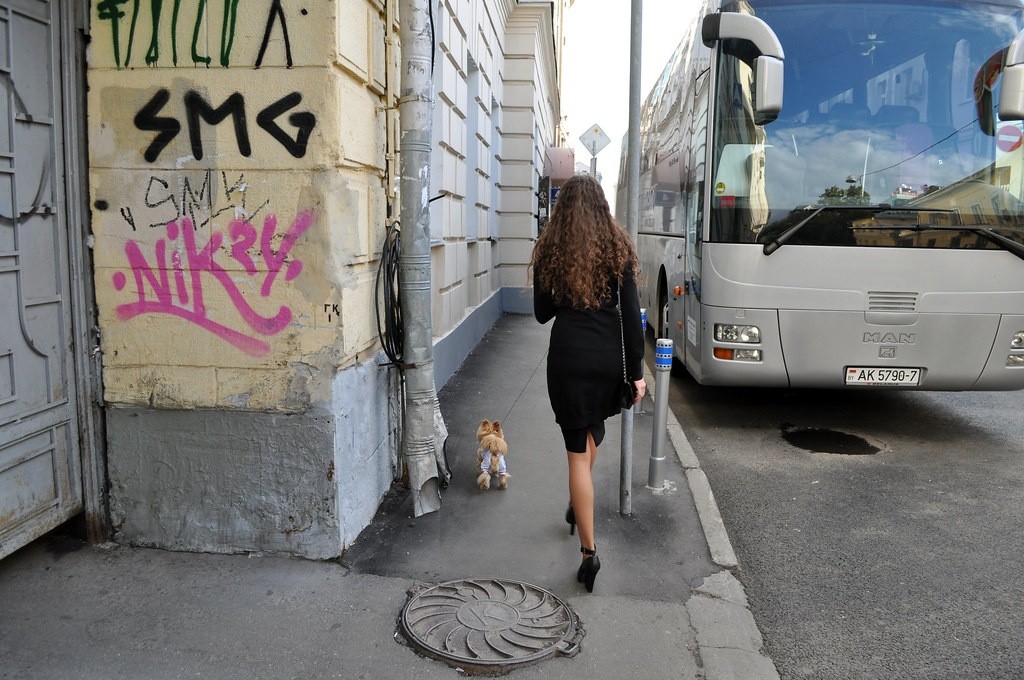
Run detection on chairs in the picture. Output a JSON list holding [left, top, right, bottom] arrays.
[[761, 99, 960, 195]]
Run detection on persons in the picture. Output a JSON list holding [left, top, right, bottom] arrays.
[[528, 174, 646, 593]]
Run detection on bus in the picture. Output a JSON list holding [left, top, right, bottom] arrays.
[[609, 0, 1024, 407]]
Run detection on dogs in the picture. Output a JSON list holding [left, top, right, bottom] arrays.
[[475, 418, 512, 492]]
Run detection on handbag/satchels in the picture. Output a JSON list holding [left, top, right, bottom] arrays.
[[620, 377, 637, 410]]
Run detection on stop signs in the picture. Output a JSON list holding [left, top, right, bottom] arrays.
[[997, 125, 1024, 152]]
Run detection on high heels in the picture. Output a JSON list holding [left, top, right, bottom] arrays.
[[565, 507, 578, 535], [576, 543, 601, 593]]
